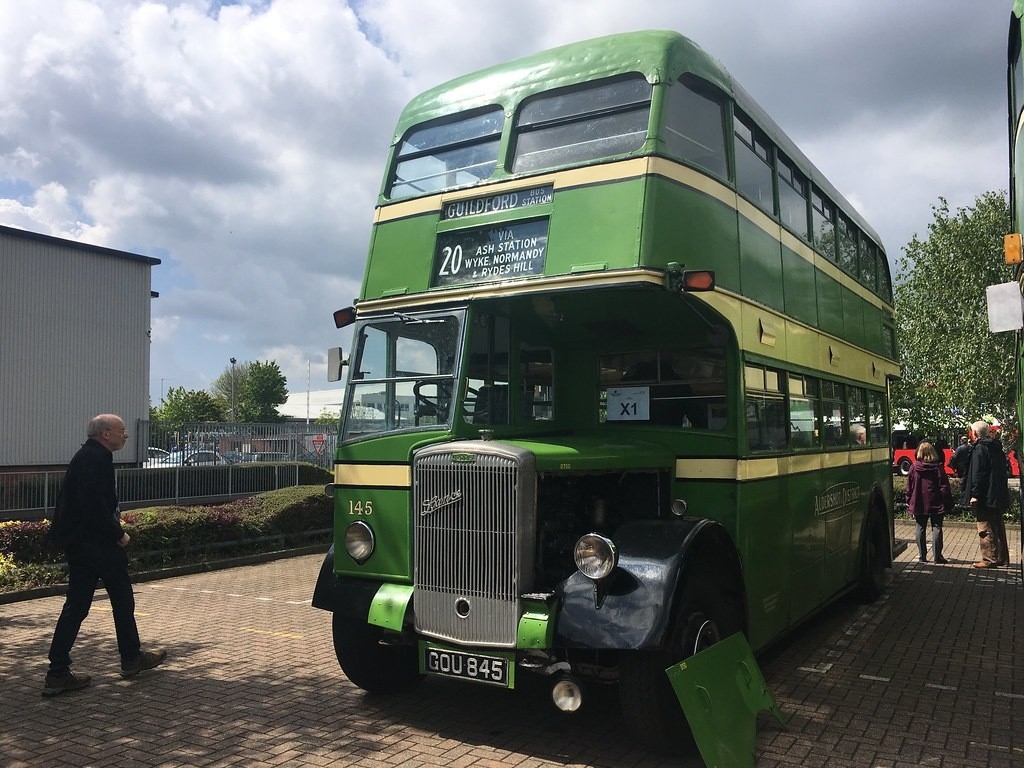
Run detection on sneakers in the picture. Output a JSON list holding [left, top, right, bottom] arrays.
[[120, 648, 167, 677], [43, 670, 91, 695]]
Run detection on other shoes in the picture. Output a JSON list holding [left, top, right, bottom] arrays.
[[973, 560, 997, 569], [936, 558, 947, 564]]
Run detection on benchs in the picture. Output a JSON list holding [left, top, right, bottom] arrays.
[[764, 202, 792, 227], [689, 153, 727, 181], [737, 181, 762, 203]]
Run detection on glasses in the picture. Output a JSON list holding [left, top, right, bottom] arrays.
[[106, 429, 127, 435]]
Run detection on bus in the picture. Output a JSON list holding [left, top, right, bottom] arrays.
[[311, 30, 911, 768], [891, 418, 1020, 479], [983, 0, 1024, 552]]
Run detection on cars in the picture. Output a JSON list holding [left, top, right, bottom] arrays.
[[220, 451, 289, 462], [148, 449, 228, 468], [143, 447, 170, 468]]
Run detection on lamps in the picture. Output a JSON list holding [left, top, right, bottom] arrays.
[[151, 291, 159, 298]]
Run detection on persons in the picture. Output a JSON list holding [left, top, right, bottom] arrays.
[[966, 421, 1012, 567], [605, 380, 708, 430], [904, 431, 954, 564], [953, 436, 975, 477], [41, 414, 168, 697], [850, 424, 867, 445]]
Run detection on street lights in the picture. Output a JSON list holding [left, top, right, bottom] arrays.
[[229, 358, 237, 436]]
